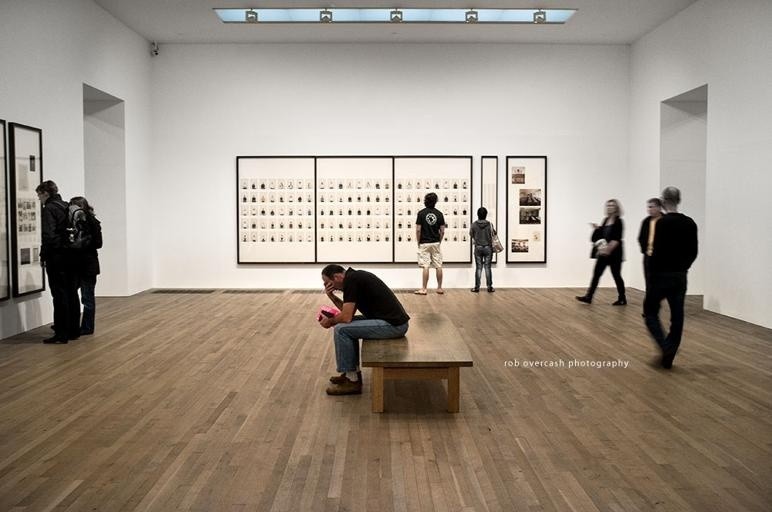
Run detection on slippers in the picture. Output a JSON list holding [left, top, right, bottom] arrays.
[[414, 289, 428, 295], [437, 288, 443, 293]]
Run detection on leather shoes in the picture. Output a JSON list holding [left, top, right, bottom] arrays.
[[330, 372, 363, 382], [79, 329, 94, 335], [488, 286, 494, 293], [43, 336, 68, 344], [471, 287, 480, 293], [325, 382, 362, 394]]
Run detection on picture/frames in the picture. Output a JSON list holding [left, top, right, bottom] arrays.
[[482, 157, 497, 264], [508, 156, 545, 263], [393, 155, 472, 263], [8, 121, 44, 297], [0, 119, 9, 300], [236, 156, 315, 262], [315, 155, 394, 263]]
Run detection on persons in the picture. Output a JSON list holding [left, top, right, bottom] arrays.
[[638, 199, 674, 318], [469, 208, 504, 292], [35, 180, 92, 344], [317, 265, 410, 395], [414, 193, 445, 295], [575, 200, 627, 305], [642, 186, 698, 369], [51, 197, 103, 335]]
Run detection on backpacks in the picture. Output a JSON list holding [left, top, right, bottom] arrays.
[[52, 200, 91, 248]]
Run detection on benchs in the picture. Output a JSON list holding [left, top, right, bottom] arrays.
[[362, 312, 473, 413]]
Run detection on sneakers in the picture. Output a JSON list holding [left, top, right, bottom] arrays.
[[575, 295, 592, 304], [611, 299, 628, 306]]
